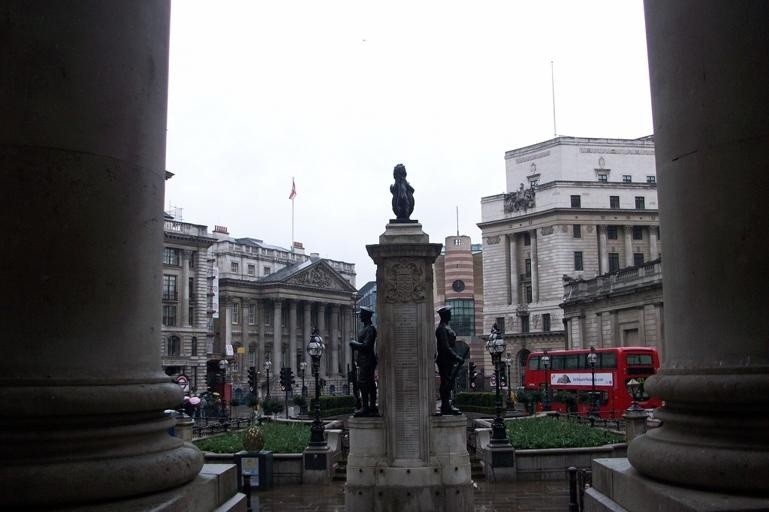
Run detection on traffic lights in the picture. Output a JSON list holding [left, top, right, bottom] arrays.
[[504, 351, 516, 411], [254, 368, 261, 388], [470, 381, 477, 389], [285, 367, 295, 391], [246, 366, 257, 387], [280, 367, 286, 386], [467, 362, 477, 380]]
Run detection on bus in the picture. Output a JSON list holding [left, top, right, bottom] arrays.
[[522, 346, 664, 428]]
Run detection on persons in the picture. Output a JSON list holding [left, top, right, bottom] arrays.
[[182, 391, 223, 420], [347, 302, 380, 417], [432, 303, 467, 417]]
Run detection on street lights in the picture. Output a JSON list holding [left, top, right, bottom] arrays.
[[263, 357, 273, 400], [586, 347, 603, 419], [305, 325, 332, 452], [623, 378, 644, 415], [215, 356, 229, 427], [541, 351, 554, 411], [299, 359, 311, 416], [482, 323, 516, 450]]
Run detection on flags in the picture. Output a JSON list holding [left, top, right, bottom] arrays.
[[288, 180, 298, 201]]
